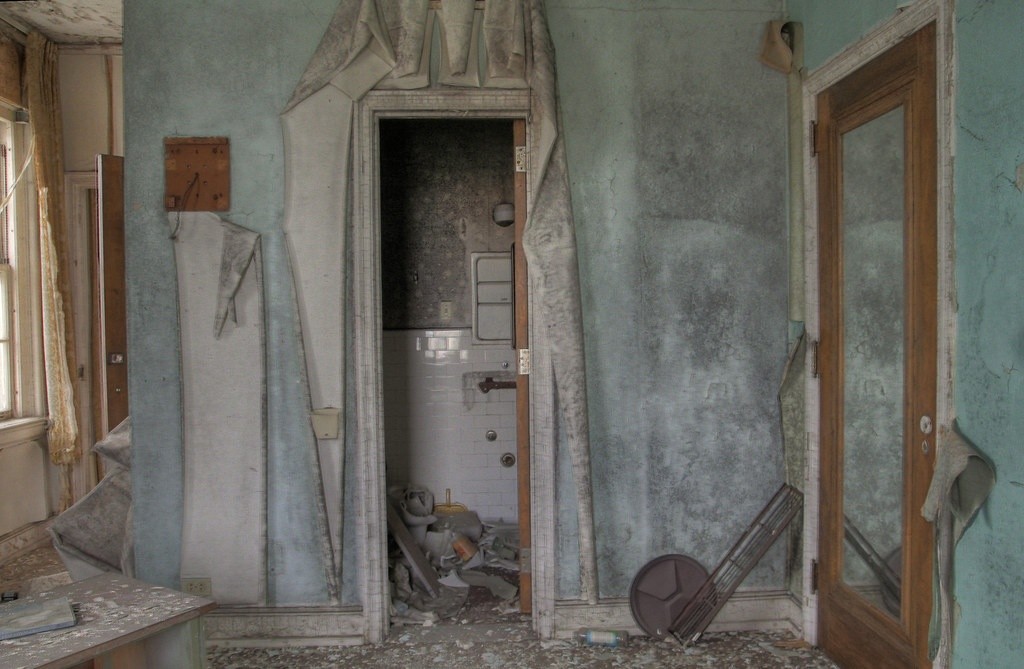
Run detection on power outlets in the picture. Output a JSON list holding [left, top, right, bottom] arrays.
[[182, 578, 211, 595]]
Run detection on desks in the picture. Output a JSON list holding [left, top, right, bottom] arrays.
[[0, 571, 218, 669]]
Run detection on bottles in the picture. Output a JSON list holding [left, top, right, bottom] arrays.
[[571, 627, 628, 647]]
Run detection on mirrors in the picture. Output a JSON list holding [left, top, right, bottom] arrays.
[[837, 83, 908, 629]]
